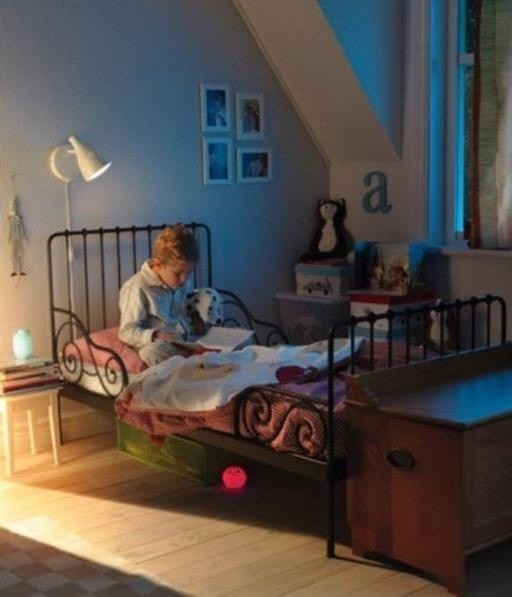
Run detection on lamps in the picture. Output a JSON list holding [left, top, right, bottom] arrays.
[[52, 133, 113, 185]]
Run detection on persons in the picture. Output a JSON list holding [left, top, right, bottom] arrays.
[[117, 225, 208, 368]]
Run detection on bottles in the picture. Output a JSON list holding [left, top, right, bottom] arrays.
[[12, 329, 33, 358]]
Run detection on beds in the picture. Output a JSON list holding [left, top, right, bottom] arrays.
[[45, 219, 508, 559]]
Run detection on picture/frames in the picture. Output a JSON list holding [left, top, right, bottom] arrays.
[[200, 79, 273, 186]]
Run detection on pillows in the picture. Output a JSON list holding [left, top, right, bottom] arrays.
[[64, 327, 148, 374]]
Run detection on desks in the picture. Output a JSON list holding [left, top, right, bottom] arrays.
[[0, 379, 68, 478]]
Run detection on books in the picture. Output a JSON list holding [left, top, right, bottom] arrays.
[[163, 326, 256, 353], [0, 356, 67, 396]]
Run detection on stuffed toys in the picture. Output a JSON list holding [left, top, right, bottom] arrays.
[[300, 198, 354, 263]]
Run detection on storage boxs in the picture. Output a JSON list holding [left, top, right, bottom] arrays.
[[275, 261, 436, 343], [117, 415, 266, 486]]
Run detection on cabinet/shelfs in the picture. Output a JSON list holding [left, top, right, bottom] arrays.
[[339, 341, 512, 567]]
